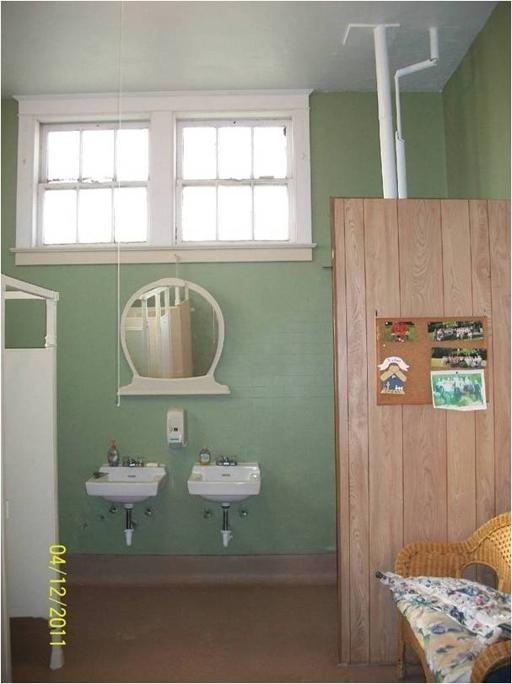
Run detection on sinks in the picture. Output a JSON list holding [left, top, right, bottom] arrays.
[[85, 463, 166, 501], [187, 462, 262, 502]]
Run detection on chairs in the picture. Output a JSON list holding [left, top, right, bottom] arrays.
[[394, 513, 510, 683]]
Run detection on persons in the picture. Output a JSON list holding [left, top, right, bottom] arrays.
[[390, 320, 411, 343], [430, 324, 482, 403]]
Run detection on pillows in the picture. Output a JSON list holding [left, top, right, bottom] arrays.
[[374, 570, 511, 636]]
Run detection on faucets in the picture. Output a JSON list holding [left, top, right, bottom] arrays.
[[223, 456, 230, 465], [128, 456, 136, 466]]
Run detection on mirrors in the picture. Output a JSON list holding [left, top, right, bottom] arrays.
[[117, 278, 232, 397]]
